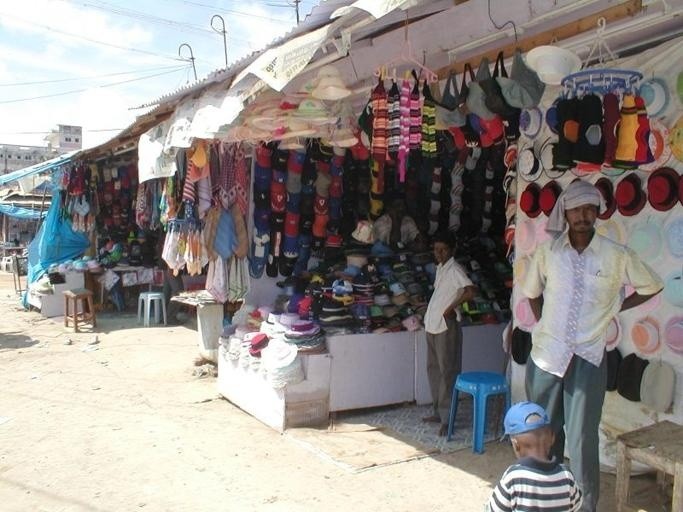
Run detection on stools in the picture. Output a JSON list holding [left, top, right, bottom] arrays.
[[136, 292, 168, 328], [62, 288, 96, 332], [446, 371, 510, 455], [612, 419, 683, 512]]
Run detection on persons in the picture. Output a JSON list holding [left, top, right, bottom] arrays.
[[421, 234, 475, 436], [483, 400, 584, 512], [156, 225, 184, 326], [524, 180, 664, 511]]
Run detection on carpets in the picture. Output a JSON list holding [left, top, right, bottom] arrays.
[[287, 397, 508, 476]]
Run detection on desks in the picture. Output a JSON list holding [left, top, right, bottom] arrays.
[[171, 289, 243, 361]]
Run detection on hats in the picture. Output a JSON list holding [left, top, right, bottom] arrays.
[[514, 70, 683, 259], [498, 400, 552, 443], [606, 276, 683, 413], [251, 131, 514, 334], [219, 324, 330, 381], [513, 259, 543, 365]]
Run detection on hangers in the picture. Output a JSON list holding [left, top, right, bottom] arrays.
[[372, 37, 441, 85]]
